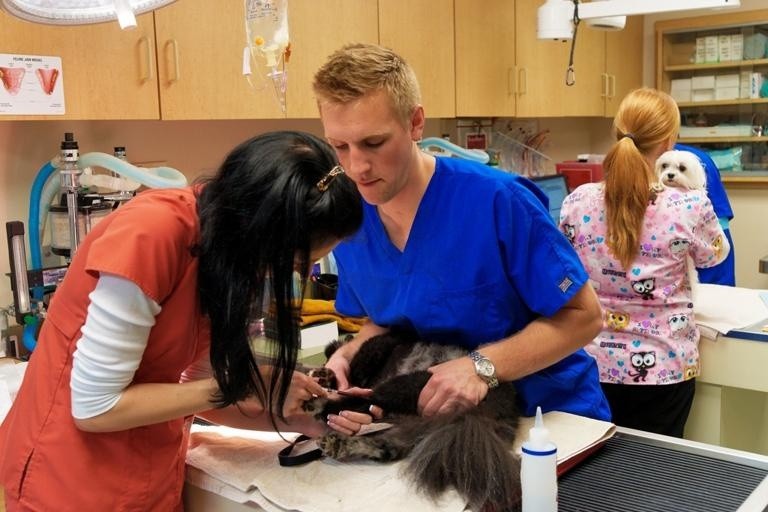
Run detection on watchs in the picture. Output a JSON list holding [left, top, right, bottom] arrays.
[[465, 350, 499, 391]]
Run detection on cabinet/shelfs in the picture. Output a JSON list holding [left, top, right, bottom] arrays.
[[654, 23, 767, 181]]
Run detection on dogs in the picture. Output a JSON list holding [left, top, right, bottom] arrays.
[[655, 151, 710, 318], [301, 333, 522, 511]]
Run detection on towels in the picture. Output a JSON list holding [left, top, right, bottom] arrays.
[[184, 408, 617, 511], [267, 298, 369, 333], [692, 283, 768, 342]]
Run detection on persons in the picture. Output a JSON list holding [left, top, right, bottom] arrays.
[[313, 41, 616, 428], [557, 87, 730, 442], [669, 143, 736, 287], [0, 129, 382, 510]]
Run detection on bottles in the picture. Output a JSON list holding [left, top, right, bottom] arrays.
[[519, 403, 561, 512]]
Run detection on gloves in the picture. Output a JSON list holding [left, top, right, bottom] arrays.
[[296, 313, 361, 333], [269, 297, 366, 323]]
